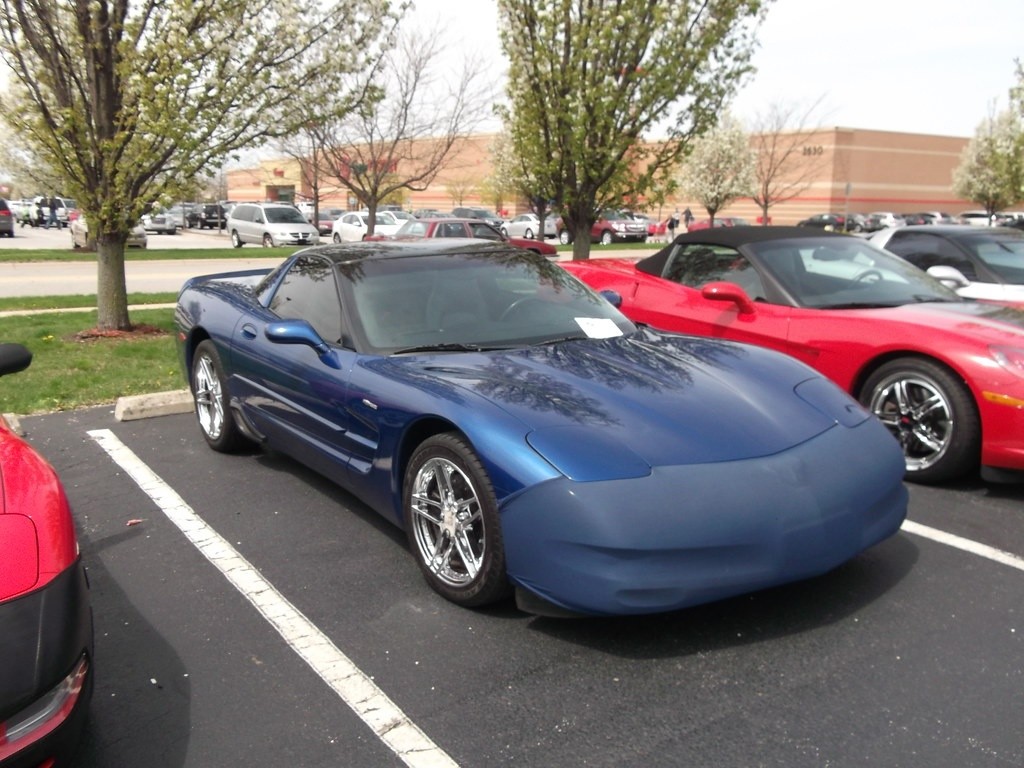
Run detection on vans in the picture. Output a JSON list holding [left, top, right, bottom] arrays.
[[297, 202, 315, 214]]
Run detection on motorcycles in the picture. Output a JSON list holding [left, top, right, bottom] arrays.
[[796, 226, 1024, 305]]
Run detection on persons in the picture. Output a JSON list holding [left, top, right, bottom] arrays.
[[20, 203, 40, 227], [45, 197, 62, 230], [682, 207, 692, 226], [662, 208, 680, 242]]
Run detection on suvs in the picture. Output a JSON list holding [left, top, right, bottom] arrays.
[[28, 196, 82, 228], [555, 207, 649, 248]]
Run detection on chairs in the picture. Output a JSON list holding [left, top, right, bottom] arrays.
[[681, 248, 724, 288], [936, 241, 961, 267], [426, 258, 495, 332], [766, 249, 803, 296]]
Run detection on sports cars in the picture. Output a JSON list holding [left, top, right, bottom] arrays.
[[539, 224, 1024, 497], [0, 342, 100, 768], [175, 240, 906, 628]]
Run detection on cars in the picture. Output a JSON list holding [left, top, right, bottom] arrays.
[[9, 200, 34, 225], [376, 201, 504, 229], [361, 218, 560, 261], [178, 201, 197, 215], [500, 213, 557, 240], [141, 202, 177, 236], [646, 219, 666, 236], [71, 212, 148, 251], [184, 203, 227, 231], [0, 198, 15, 237], [331, 211, 404, 243], [304, 212, 335, 237], [686, 216, 749, 233], [225, 203, 321, 249], [801, 208, 1023, 232]]
